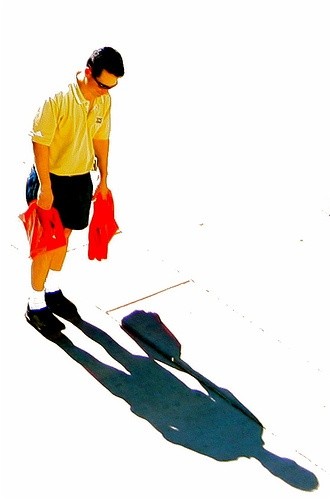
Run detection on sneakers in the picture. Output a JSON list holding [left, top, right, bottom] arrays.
[[24, 303, 65, 334], [44, 289, 78, 317]]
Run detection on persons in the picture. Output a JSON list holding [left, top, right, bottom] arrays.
[[23, 46, 126, 334]]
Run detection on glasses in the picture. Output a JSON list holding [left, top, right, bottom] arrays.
[[91, 71, 118, 90]]
